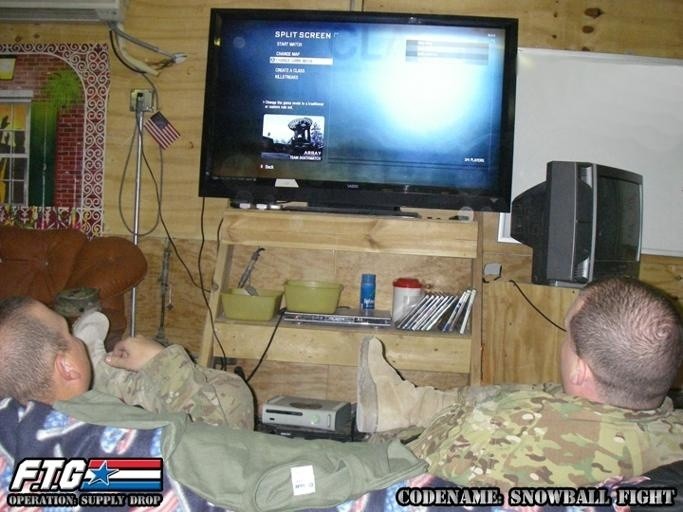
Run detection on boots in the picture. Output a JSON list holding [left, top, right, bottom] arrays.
[[357, 336, 459, 432], [71, 311, 109, 368]]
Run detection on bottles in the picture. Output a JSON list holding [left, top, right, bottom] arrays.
[[390, 277, 422, 320], [359, 273, 376, 310]]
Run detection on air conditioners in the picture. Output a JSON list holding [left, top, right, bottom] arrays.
[[0, 0, 126, 23]]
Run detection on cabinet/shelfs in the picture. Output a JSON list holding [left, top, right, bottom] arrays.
[[193, 211, 482, 420], [483, 277, 587, 395]]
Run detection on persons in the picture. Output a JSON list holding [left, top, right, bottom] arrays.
[[354, 274, 682, 512], [0, 296, 257, 433]]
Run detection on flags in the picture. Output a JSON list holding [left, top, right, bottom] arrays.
[[142, 111, 181, 150]]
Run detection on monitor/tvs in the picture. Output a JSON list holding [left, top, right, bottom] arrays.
[[509, 160, 642, 287], [197, 8, 519, 218]]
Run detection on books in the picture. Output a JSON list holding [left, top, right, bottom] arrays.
[[393, 288, 475, 336], [281, 306, 389, 327]]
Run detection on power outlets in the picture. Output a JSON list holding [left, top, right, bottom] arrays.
[[130, 88, 154, 112]]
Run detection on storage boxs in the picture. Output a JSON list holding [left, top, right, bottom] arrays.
[[283, 278, 344, 315], [221, 287, 283, 322]]
